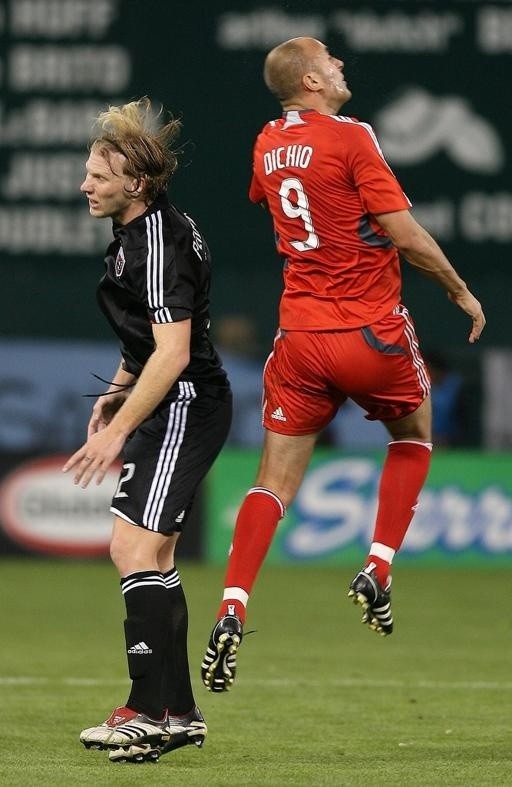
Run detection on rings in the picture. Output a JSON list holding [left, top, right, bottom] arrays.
[[84, 456, 92, 461]]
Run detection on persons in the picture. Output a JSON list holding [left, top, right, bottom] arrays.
[[201, 35, 487, 693], [422, 349, 471, 449], [217, 313, 262, 443], [59, 95, 233, 764]]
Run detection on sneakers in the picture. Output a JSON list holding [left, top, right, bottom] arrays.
[[202, 614, 243, 695], [348, 568, 395, 638], [77, 707, 171, 752], [107, 705, 207, 762]]
